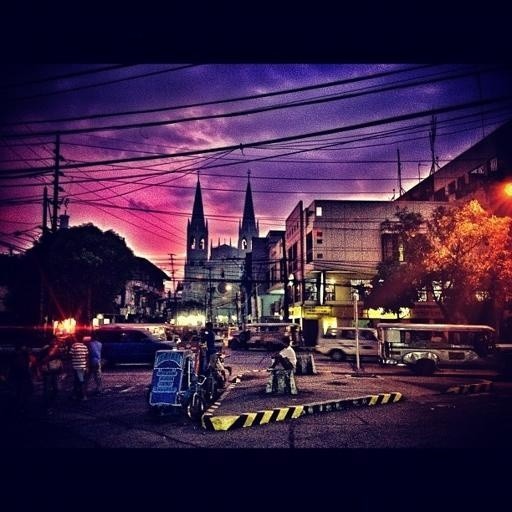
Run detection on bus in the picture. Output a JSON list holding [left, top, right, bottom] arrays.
[[229, 322, 302, 350], [379, 323, 511, 374]]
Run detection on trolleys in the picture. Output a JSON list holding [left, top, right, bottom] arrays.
[[148, 336, 232, 422]]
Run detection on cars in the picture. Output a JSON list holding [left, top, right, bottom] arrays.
[[0, 324, 54, 376], [91, 321, 228, 372]]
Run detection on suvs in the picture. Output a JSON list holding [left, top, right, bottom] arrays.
[[317, 327, 378, 361]]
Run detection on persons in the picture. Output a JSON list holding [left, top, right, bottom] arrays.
[[269, 337, 297, 369], [201, 323, 215, 367], [30, 335, 104, 410]]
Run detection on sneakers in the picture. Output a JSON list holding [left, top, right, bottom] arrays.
[[81, 391, 100, 403]]
